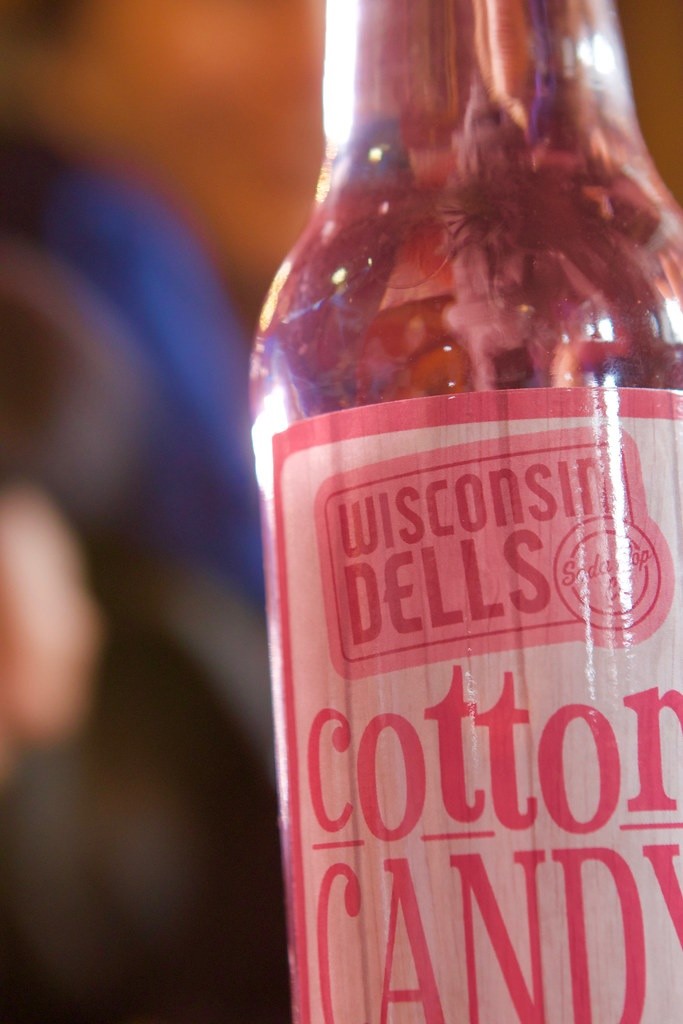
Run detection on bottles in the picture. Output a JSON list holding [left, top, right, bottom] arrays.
[[243, 2, 683, 1023]]
